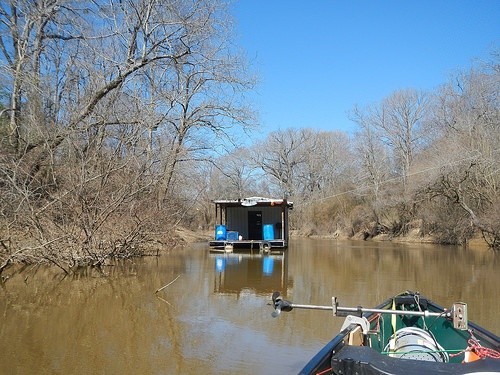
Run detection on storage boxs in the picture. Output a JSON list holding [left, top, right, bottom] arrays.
[[262, 223, 275, 241], [215, 256, 226, 272], [225, 257, 239, 264], [215, 224, 227, 240], [262, 257, 274, 274], [226, 231, 239, 241]]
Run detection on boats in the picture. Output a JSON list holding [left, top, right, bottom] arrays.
[[296, 291, 500, 375]]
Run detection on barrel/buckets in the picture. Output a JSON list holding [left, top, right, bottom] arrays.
[[263, 223, 274, 241], [215, 255, 226, 272], [215, 225, 227, 240], [262, 257, 274, 278]]
